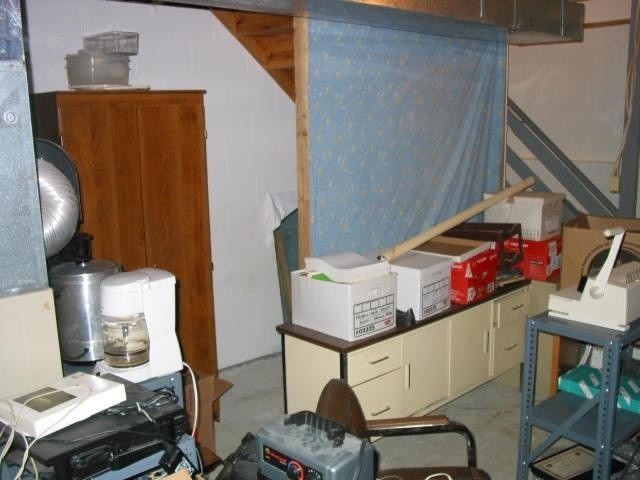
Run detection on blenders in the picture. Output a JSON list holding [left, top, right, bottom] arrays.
[[92, 268, 184, 384]]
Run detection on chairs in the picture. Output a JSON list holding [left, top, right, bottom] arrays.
[[316, 378, 491, 480]]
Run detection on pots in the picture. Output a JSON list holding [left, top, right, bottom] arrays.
[[47, 255, 124, 364]]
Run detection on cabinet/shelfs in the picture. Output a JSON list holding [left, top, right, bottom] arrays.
[[34, 89, 221, 423], [516, 310, 639, 479], [275, 278, 531, 444]]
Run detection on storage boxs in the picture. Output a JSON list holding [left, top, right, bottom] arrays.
[[184, 370, 234, 467]]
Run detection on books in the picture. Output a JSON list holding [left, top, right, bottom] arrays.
[[0, 371, 126, 438]]
